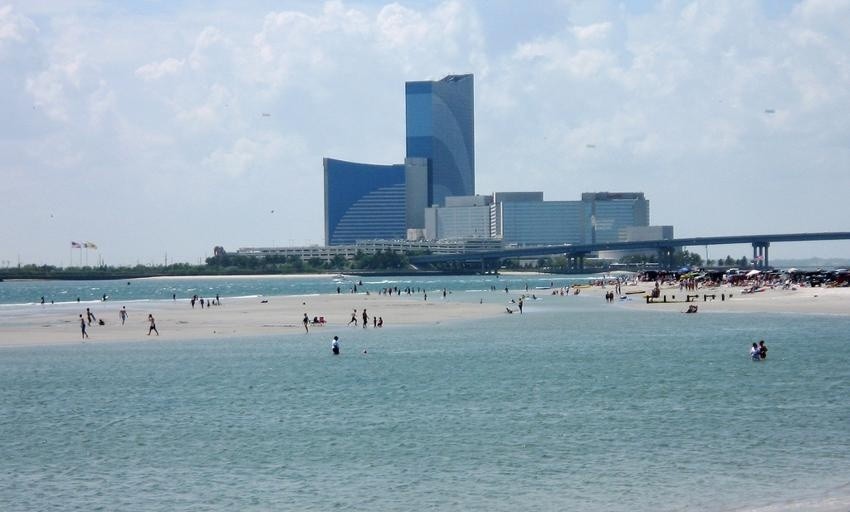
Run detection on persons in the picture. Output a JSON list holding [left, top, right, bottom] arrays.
[[337, 280, 452, 301], [80, 308, 104, 339], [480, 283, 537, 314], [190, 293, 221, 308], [172, 293, 176, 300], [303, 309, 383, 355], [750, 340, 768, 361], [589, 275, 634, 301], [146, 314, 159, 336], [636, 269, 850, 299], [551, 281, 581, 296], [119, 306, 129, 326]]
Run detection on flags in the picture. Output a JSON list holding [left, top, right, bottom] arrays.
[[70, 240, 97, 248]]
